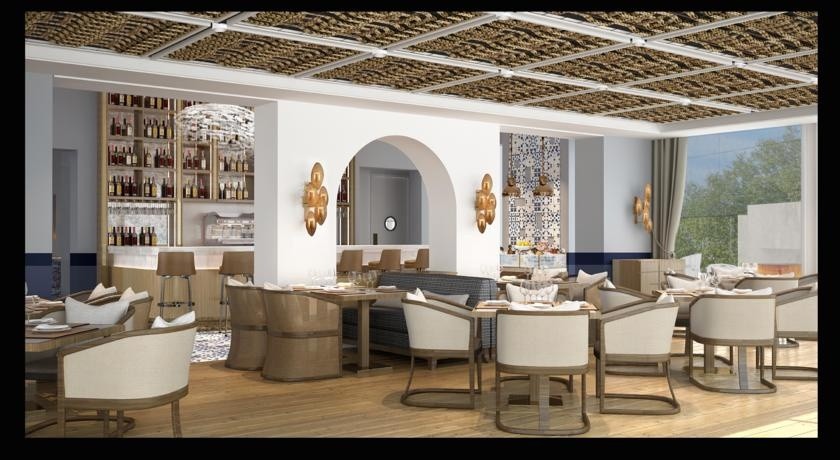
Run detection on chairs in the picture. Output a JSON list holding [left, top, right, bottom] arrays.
[[61, 273, 818, 437]]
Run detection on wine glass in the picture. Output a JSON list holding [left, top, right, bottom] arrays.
[[348, 271, 362, 292], [363, 273, 371, 291], [534, 280, 554, 305], [25, 296, 35, 331], [369, 270, 377, 290], [520, 281, 534, 305], [312, 268, 335, 288], [699, 272, 718, 294]]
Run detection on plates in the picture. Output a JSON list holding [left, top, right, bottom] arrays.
[[26, 319, 71, 332]]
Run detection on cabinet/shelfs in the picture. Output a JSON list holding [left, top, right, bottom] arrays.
[[97, 92, 354, 287], [107, 245, 255, 331], [612, 259, 686, 294]]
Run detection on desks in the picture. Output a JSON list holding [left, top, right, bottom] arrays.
[[25, 324, 136, 438], [471, 300, 599, 408], [295, 287, 407, 374]]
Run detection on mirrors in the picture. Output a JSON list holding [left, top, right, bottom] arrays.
[[384, 216, 397, 231]]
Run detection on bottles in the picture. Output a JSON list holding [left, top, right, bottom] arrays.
[[144, 176, 173, 197], [108, 145, 137, 167], [144, 146, 173, 168], [108, 92, 196, 110], [116, 226, 137, 246], [219, 153, 249, 172], [107, 176, 137, 196], [110, 118, 133, 137], [184, 178, 205, 198], [144, 118, 173, 139], [139, 227, 157, 246], [108, 225, 116, 246], [219, 179, 249, 200], [183, 150, 207, 171]]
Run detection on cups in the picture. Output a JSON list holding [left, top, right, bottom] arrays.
[[211, 224, 254, 238], [742, 262, 757, 273], [48, 314, 56, 325]]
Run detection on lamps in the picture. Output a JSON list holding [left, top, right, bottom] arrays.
[[474, 174, 496, 233], [303, 163, 328, 236], [633, 183, 653, 232], [502, 133, 553, 197]]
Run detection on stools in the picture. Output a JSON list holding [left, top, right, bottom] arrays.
[[369, 249, 401, 272], [155, 252, 197, 320], [337, 249, 362, 272], [217, 251, 254, 337], [404, 249, 429, 272]]
